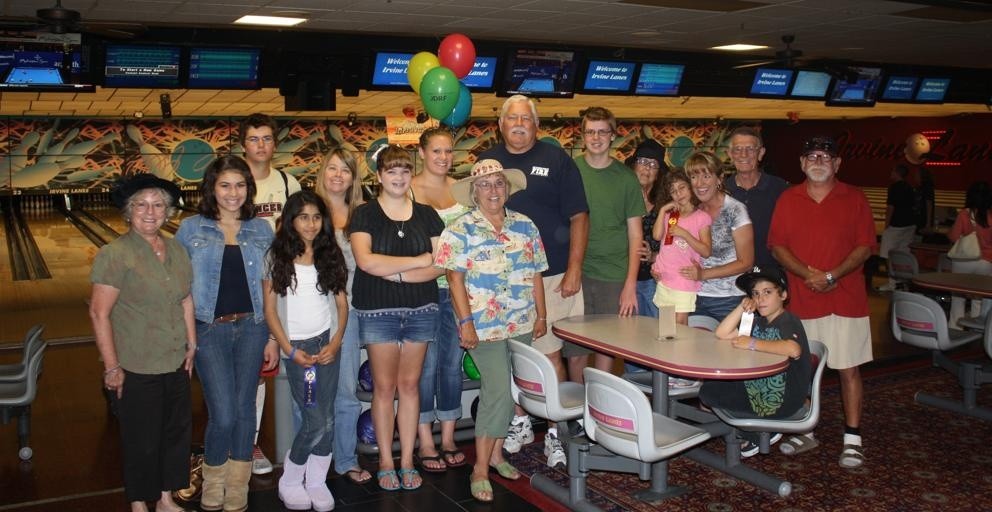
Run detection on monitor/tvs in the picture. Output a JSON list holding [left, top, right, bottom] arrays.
[[748, 65, 951, 108], [97, 42, 183, 88], [582, 58, 638, 96], [371, 50, 499, 91], [631, 61, 686, 96], [498, 42, 575, 99], [1, 35, 96, 93], [185, 44, 260, 90]]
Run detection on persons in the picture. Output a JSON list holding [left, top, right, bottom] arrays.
[[947, 179, 992, 332]]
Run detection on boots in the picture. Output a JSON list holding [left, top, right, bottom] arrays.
[[277, 448, 313, 511], [304, 452, 336, 512], [200, 457, 228, 511], [223, 457, 253, 512]]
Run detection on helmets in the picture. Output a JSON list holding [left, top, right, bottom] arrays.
[[903, 133, 931, 166]]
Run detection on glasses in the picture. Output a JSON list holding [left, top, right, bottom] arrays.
[[635, 158, 661, 169], [803, 152, 835, 163], [582, 128, 613, 137], [730, 144, 759, 155], [243, 136, 273, 146], [473, 178, 507, 191]]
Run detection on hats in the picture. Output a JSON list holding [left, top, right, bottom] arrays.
[[448, 158, 529, 209], [735, 265, 789, 298], [624, 138, 670, 176], [800, 130, 837, 157], [108, 173, 181, 210]]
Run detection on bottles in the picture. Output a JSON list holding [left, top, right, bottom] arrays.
[[303, 363, 318, 407]]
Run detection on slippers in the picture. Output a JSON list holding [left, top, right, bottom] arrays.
[[838, 443, 865, 469], [470, 474, 494, 503], [397, 469, 423, 491], [415, 449, 448, 473], [376, 470, 402, 491], [438, 444, 467, 468], [489, 462, 522, 482], [779, 434, 820, 457], [344, 468, 372, 485]]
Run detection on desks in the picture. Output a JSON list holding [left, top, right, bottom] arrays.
[[550, 310, 793, 501]]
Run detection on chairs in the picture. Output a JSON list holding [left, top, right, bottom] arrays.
[[505, 338, 586, 423], [0, 340, 49, 462], [711, 337, 830, 436], [0, 319, 47, 423], [582, 366, 713, 465], [622, 313, 722, 399], [884, 247, 992, 422]]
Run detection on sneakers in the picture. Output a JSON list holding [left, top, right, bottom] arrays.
[[568, 420, 586, 439], [501, 419, 536, 457], [250, 447, 274, 476], [543, 432, 567, 470], [739, 431, 784, 459]]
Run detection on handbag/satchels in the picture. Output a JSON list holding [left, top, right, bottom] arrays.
[[177, 451, 206, 504], [946, 207, 982, 259]]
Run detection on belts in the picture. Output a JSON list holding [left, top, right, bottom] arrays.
[[214, 312, 251, 323]]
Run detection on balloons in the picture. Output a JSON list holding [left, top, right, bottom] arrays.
[[438, 33, 476, 80], [419, 66, 460, 121], [407, 51, 441, 94], [441, 79, 472, 128]]
